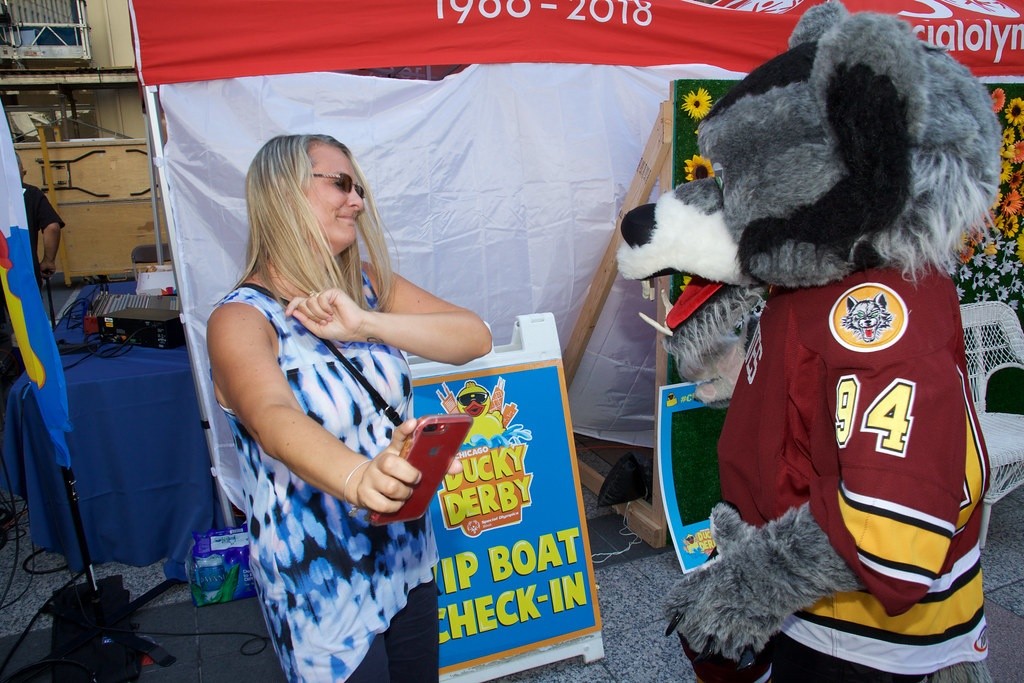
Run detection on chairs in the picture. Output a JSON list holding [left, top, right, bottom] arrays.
[[958, 301, 1024, 547]]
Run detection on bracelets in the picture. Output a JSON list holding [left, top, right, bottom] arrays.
[[342, 459, 372, 517]]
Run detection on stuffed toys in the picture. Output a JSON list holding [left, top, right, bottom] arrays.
[[616, 6, 1001, 683]]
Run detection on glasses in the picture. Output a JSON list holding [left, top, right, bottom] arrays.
[[306, 172, 365, 198]]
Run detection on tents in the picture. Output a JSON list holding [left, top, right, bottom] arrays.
[[129, 0, 1024, 530]]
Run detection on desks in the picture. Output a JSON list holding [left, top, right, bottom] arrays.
[[0, 281, 230, 582]]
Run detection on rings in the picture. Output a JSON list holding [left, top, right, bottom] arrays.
[[309, 291, 320, 297]]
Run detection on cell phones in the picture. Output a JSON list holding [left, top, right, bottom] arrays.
[[366, 413, 474, 526]]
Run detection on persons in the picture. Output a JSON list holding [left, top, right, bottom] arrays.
[[205, 134, 493, 683], [0, 152, 65, 381]]
